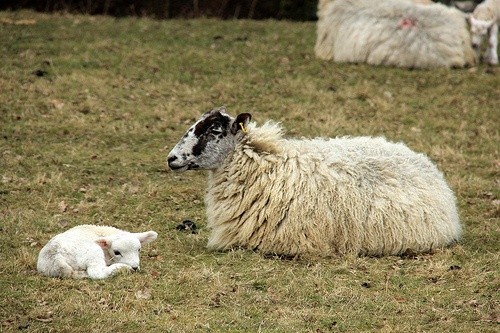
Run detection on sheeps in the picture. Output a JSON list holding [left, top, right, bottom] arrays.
[[166, 106, 464, 259], [36, 224, 158, 281], [465, 0, 500, 66], [314, 0, 477, 70]]
